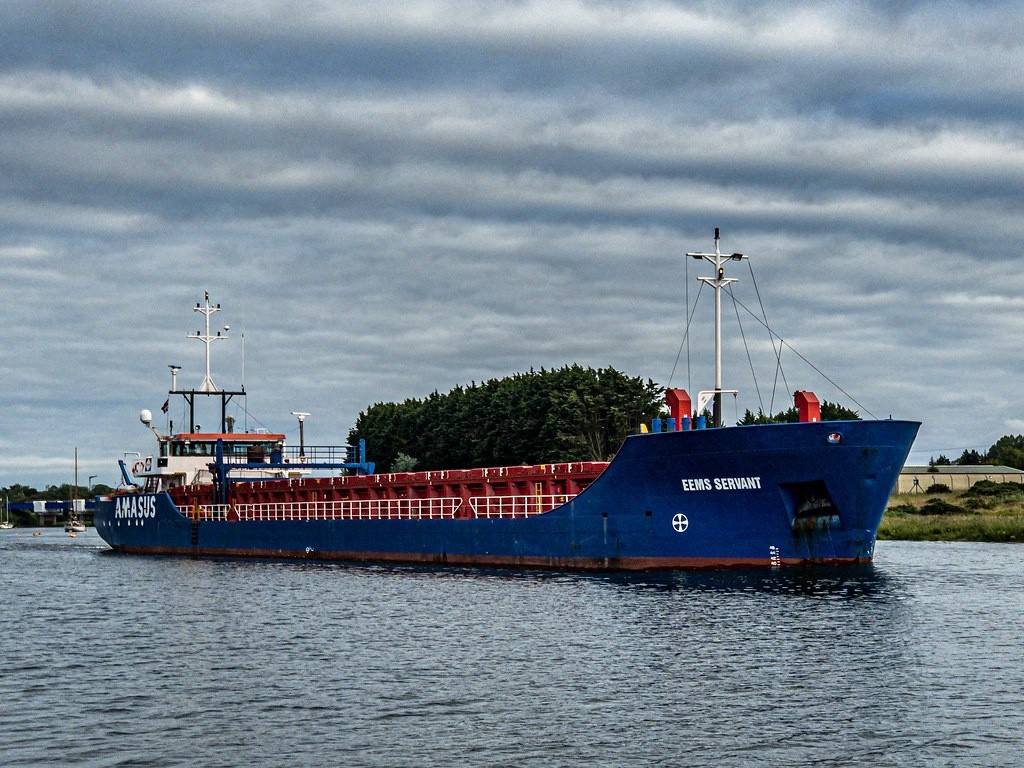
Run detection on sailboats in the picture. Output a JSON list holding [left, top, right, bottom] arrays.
[[0, 495, 14, 529], [63, 445, 87, 532]]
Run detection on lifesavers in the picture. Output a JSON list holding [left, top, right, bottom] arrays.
[[133, 461, 144, 473], [169, 482, 175, 489], [300, 456, 305, 462]]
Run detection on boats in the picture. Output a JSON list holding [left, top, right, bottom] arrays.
[[93, 227, 925, 578]]
